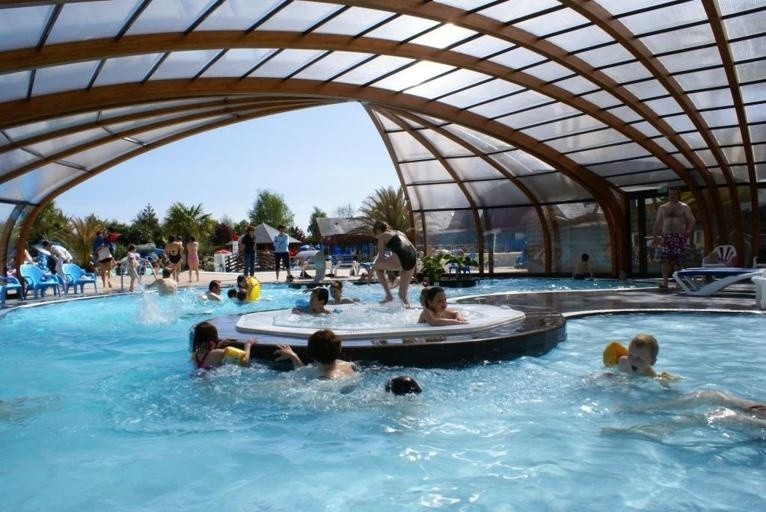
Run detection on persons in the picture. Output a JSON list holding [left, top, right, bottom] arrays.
[[289, 246, 299, 259], [206, 280, 221, 301], [306, 244, 360, 275], [651, 186, 696, 289], [2, 236, 74, 297], [292, 281, 354, 313], [273, 225, 290, 280], [228, 275, 247, 301], [417, 287, 470, 326], [598, 390, 766, 438], [368, 220, 417, 304], [592, 333, 670, 392], [575, 253, 594, 281], [240, 226, 256, 276], [87, 228, 200, 297], [262, 247, 272, 266], [189, 321, 422, 398]]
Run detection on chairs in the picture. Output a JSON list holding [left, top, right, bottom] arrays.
[[61, 263, 98, 295], [701, 244, 737, 269], [673, 267, 766, 296], [20, 263, 61, 298], [449, 252, 476, 273], [0, 275, 24, 301]]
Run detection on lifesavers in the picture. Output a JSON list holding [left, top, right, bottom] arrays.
[[244, 274, 259, 303], [603, 238, 662, 270], [332, 307, 342, 313], [295, 299, 309, 313], [603, 341, 629, 368], [220, 346, 244, 366]]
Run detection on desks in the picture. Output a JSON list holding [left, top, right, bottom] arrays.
[[751, 275, 766, 310]]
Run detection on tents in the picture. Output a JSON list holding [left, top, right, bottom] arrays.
[[238, 222, 302, 243]]
